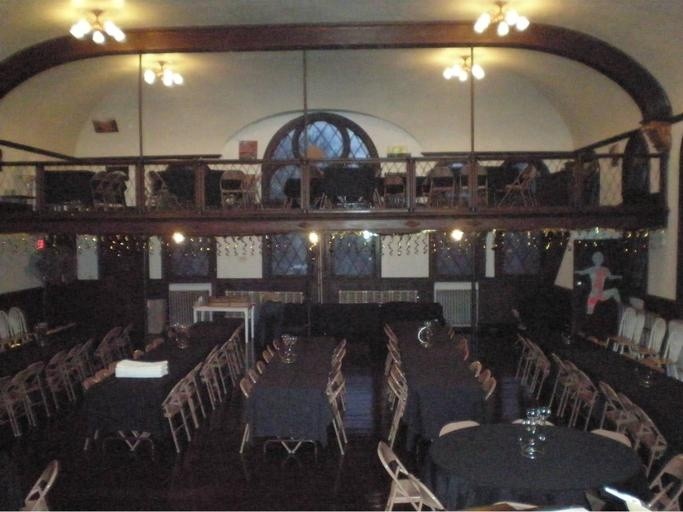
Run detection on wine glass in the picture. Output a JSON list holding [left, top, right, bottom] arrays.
[[514, 404, 552, 459], [422, 317, 439, 352], [173, 324, 187, 350], [279, 333, 297, 363]]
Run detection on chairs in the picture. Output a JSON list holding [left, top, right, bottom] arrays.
[[377, 442, 446, 512], [18, 460, 58, 512], [147, 161, 266, 211], [91, 169, 129, 211], [1, 307, 28, 342], [283, 156, 601, 211], [380, 316, 498, 450], [1, 323, 135, 437], [238, 335, 350, 455], [512, 306, 683, 511], [82, 318, 244, 455]]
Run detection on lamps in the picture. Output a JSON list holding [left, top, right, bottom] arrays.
[[143, 59, 184, 88], [442, 52, 484, 82], [68, 9, 127, 45], [474, 0, 534, 38]]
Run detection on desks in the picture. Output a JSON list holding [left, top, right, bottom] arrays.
[[192, 301, 255, 341]]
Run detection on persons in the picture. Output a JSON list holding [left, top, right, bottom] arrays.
[[575, 251, 622, 319]]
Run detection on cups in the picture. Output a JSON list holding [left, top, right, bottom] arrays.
[[637, 374, 656, 389]]
